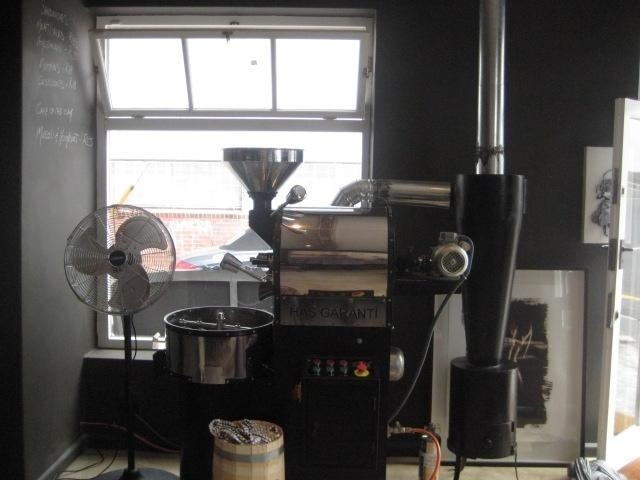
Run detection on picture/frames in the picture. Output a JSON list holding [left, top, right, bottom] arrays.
[[428, 267, 588, 468]]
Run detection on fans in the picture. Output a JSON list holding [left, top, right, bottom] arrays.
[[63, 204, 182, 480]]
